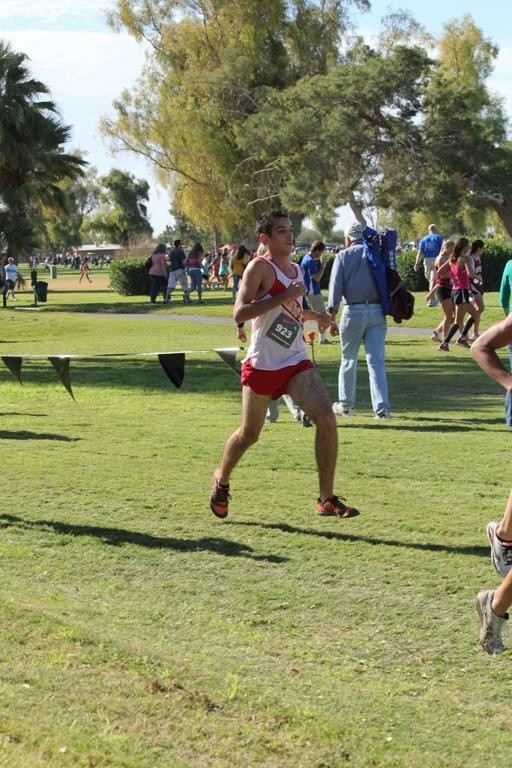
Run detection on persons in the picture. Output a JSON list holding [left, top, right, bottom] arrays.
[[184, 242, 208, 304], [469, 313, 512, 654], [148, 244, 171, 304], [326, 222, 390, 420], [499, 260, 512, 426], [236, 243, 311, 424], [78, 258, 92, 284], [467, 239, 484, 341], [4, 257, 18, 301], [299, 240, 332, 344], [231, 245, 249, 302], [425, 240, 455, 342], [32, 254, 110, 270], [201, 253, 229, 290], [209, 209, 359, 519], [435, 237, 479, 352], [162, 240, 192, 304], [414, 224, 443, 307]]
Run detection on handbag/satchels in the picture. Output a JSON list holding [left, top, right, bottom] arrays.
[[385, 266, 414, 324]]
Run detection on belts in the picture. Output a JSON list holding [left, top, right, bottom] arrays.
[[145, 254, 153, 268], [343, 300, 380, 305]]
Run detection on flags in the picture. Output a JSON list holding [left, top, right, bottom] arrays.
[[157, 353, 185, 389], [214, 349, 241, 377], [49, 357, 75, 401], [1, 356, 22, 386]]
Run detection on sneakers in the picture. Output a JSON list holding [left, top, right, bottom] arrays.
[[13, 298, 18, 301], [437, 343, 450, 353], [373, 410, 389, 420], [431, 331, 442, 343], [183, 294, 187, 303], [469, 334, 479, 341], [299, 409, 312, 427], [427, 302, 433, 307], [209, 470, 231, 518], [320, 339, 332, 345], [331, 401, 354, 416], [488, 521, 512, 578], [475, 589, 510, 655], [455, 336, 471, 348], [316, 494, 360, 517]]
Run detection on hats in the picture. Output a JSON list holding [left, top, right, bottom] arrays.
[[428, 224, 437, 230], [344, 221, 362, 241], [8, 257, 15, 261]]
